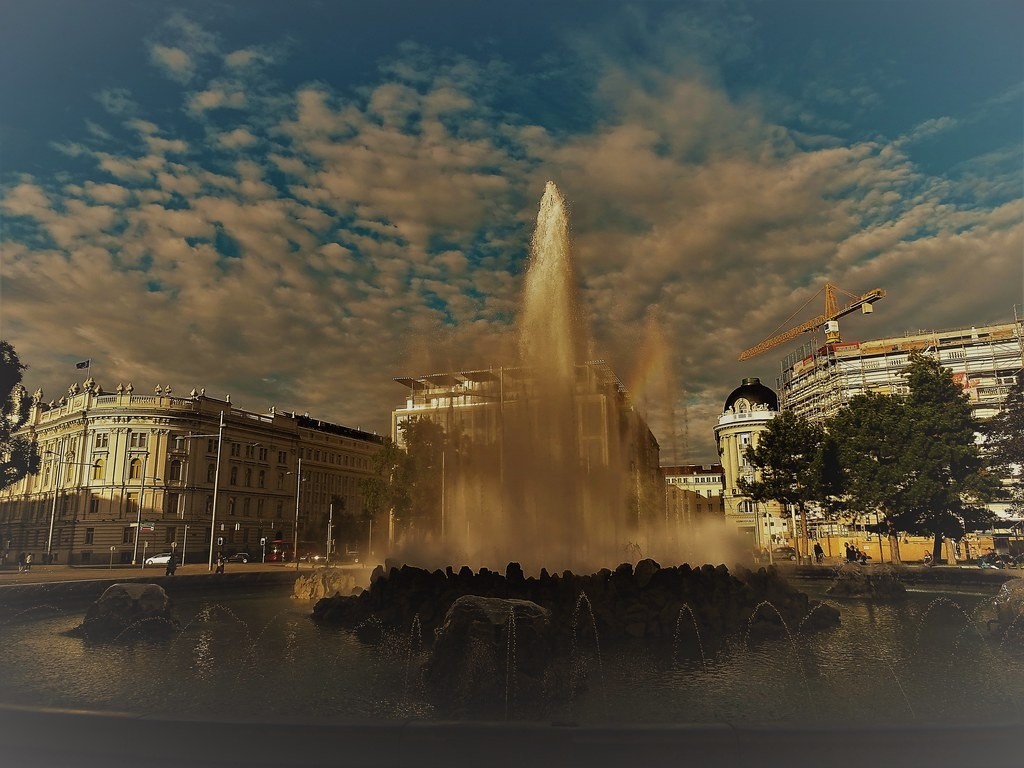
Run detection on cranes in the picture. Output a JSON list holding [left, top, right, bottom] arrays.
[[738, 283, 886, 361]]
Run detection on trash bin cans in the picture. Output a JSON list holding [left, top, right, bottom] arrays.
[[803, 556, 811, 565]]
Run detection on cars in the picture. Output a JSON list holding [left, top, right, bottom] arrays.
[[761, 547, 800, 561], [146, 553, 178, 565], [223, 540, 337, 564]]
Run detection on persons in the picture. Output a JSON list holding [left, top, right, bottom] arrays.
[[924, 550, 934, 567], [814, 543, 823, 562], [214, 550, 226, 574], [844, 542, 866, 565], [18, 552, 25, 572], [25, 552, 33, 571], [165, 552, 177, 576]]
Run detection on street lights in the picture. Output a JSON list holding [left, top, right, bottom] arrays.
[[132, 452, 161, 566], [42, 439, 101, 555], [175, 409, 263, 571], [286, 458, 307, 559]]
